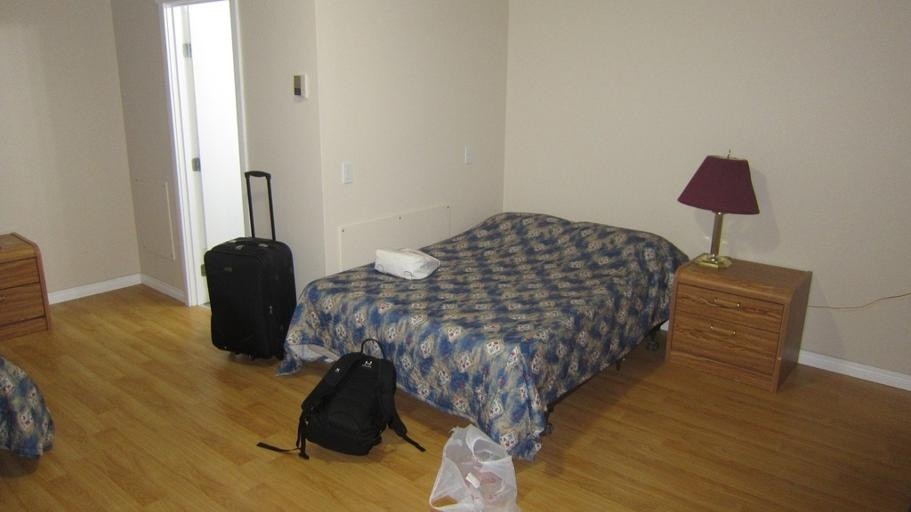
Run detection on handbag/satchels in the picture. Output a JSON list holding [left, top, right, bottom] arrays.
[[374, 249, 439, 280]]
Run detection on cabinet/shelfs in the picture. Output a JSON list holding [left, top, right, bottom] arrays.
[[1, 231, 53, 346]]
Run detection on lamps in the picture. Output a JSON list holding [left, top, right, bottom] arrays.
[[677, 147, 760, 270]]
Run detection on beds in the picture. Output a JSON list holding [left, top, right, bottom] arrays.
[[289, 208, 688, 445]]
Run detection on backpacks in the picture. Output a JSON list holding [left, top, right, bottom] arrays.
[[256, 339, 425, 458]]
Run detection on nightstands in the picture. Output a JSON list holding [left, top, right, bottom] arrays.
[[665, 253, 813, 395]]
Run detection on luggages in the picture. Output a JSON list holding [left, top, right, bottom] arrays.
[[203, 171, 296, 360]]
